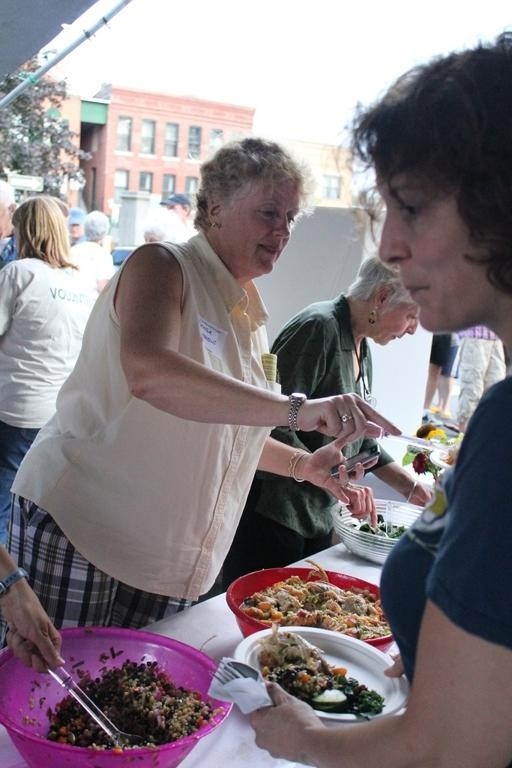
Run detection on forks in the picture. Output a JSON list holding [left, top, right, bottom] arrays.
[[208, 659, 241, 687]]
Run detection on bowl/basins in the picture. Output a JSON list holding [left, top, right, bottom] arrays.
[[226, 567, 396, 653], [0, 626, 234, 768], [229, 626, 411, 727], [430, 446, 452, 472], [330, 497, 425, 565]]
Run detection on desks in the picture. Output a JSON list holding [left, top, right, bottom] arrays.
[[1, 539, 409, 768]]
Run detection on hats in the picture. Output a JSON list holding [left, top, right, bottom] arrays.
[[67, 207, 84, 226], [159, 193, 190, 207]]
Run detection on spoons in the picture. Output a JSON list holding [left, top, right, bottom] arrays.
[[34, 653, 146, 749], [381, 429, 465, 461], [353, 508, 394, 540], [224, 661, 263, 687]]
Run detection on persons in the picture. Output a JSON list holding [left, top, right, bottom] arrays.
[[0, 195, 99, 544], [70, 210, 118, 271], [1, 546, 65, 671], [1, 214, 20, 261], [165, 191, 192, 216], [141, 217, 176, 245], [446, 327, 504, 425], [1, 138, 402, 655], [64, 209, 89, 243], [417, 332, 454, 412], [243, 35, 511, 768], [226, 254, 422, 580]]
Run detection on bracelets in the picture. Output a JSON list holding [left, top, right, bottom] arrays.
[[288, 448, 307, 483]]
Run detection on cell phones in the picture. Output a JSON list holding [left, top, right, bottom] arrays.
[[330, 444, 380, 478]]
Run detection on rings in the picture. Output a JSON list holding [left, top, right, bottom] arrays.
[[341, 413, 354, 425]]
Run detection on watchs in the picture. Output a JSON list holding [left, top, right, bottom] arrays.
[[289, 389, 308, 433], [0, 565, 31, 596]]
